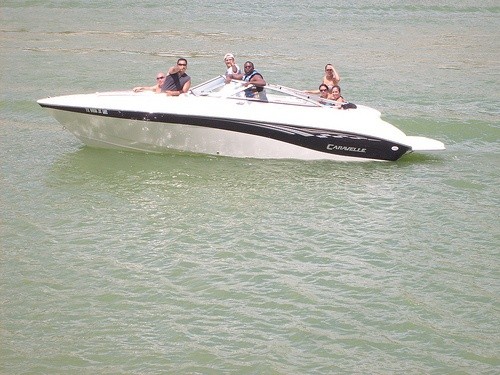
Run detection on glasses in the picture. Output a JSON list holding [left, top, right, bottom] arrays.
[[332, 91, 339, 94], [321, 90, 327, 92], [245, 66, 252, 69], [178, 64, 186, 66], [327, 69, 333, 71], [157, 77, 165, 80]]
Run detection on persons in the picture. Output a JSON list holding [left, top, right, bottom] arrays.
[[302, 64, 340, 97], [223, 53, 241, 76], [162, 58, 191, 96], [318, 84, 333, 100], [133, 66, 179, 92], [224, 61, 266, 98], [330, 87, 347, 110]]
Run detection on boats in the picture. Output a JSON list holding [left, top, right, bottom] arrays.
[[35, 72, 446, 167]]
